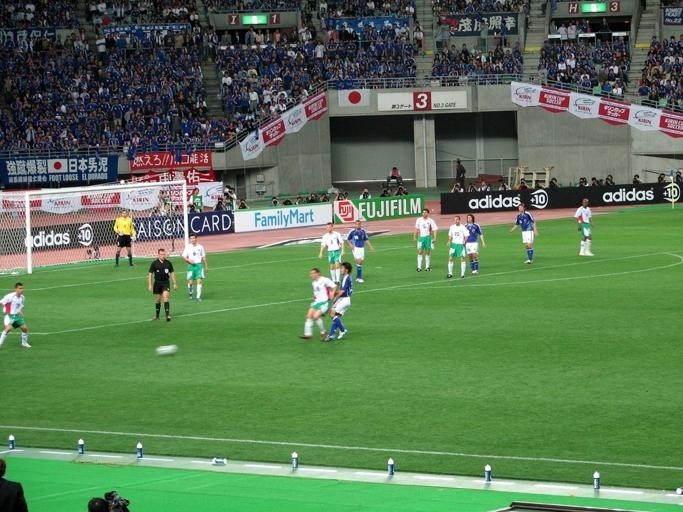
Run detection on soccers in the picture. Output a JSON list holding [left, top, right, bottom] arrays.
[[159, 343, 176, 357]]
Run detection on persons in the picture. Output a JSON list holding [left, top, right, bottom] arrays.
[[1, 1, 682, 161], [1, 282, 30, 347], [298, 220, 373, 342], [510, 203, 538, 264], [0, 459, 29, 512], [451, 158, 682, 210], [88, 497, 112, 512], [86, 189, 209, 322], [189, 166, 409, 213], [413, 209, 485, 279], [575, 198, 594, 256]]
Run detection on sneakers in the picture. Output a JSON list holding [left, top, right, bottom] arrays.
[[22, 343, 32, 348], [320, 329, 348, 342], [579, 250, 595, 256], [166, 316, 171, 321]]
